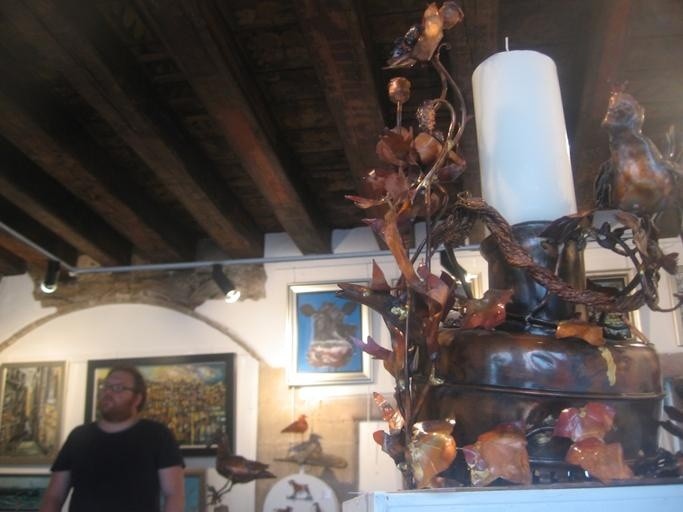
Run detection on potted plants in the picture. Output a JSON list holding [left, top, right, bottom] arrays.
[[160, 468, 207, 512], [666, 265, 682, 347], [454, 271, 482, 301], [288, 278, 375, 387], [581, 269, 642, 342], [84, 352, 236, 456], [0, 360, 68, 466]]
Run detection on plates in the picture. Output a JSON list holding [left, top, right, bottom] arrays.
[[334, 481, 682, 512]]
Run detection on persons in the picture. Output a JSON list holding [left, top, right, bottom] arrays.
[[590, 91, 674, 215], [37, 366, 187, 511]]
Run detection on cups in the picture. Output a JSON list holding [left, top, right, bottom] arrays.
[[98, 381, 135, 393]]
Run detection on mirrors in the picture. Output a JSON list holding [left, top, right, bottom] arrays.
[[289, 434, 322, 455], [206, 431, 277, 504], [280, 414, 308, 433]]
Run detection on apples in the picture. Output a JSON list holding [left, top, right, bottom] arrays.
[[470, 36, 577, 223]]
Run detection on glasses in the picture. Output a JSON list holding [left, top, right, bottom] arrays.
[[211, 264, 236, 299], [40, 258, 76, 289]]
